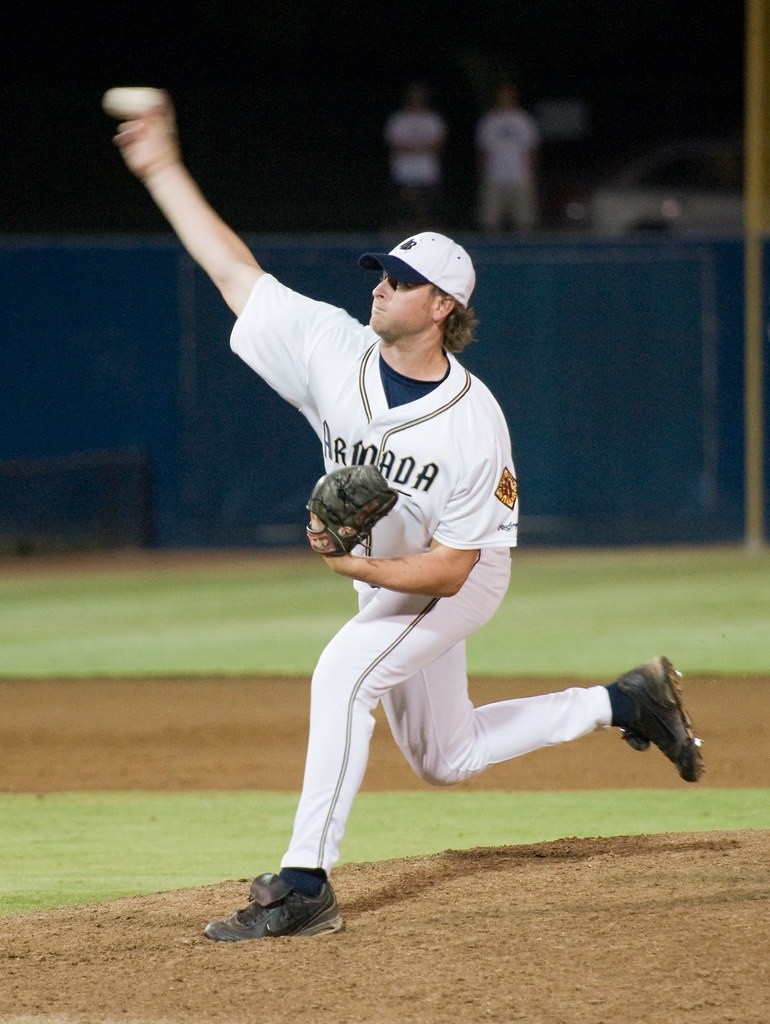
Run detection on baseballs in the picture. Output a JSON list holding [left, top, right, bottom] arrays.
[[102, 85, 167, 120]]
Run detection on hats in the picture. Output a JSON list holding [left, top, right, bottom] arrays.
[[358, 231, 476, 308]]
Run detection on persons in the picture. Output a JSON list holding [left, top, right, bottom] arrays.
[[385, 89, 446, 229], [474, 85, 540, 236], [117, 105, 704, 942]]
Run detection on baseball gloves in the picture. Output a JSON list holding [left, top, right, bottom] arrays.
[[305, 464, 399, 558]]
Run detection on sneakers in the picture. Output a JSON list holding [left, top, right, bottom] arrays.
[[618, 654, 705, 782], [205, 871, 343, 942]]
